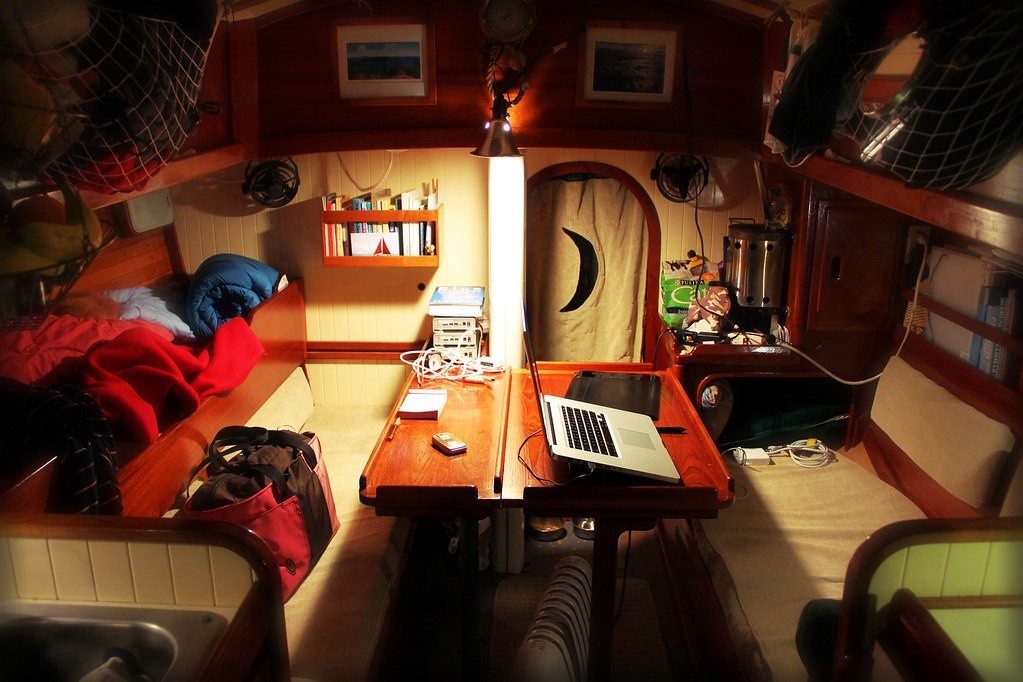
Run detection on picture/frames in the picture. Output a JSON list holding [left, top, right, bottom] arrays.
[[571, 19, 686, 113], [326, 13, 437, 107]]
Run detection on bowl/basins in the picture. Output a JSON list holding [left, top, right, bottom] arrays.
[[572, 517, 594, 539], [529, 516, 565, 541]]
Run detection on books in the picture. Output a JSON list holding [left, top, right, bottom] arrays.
[[321, 188, 437, 256], [398, 389, 448, 420], [920, 241, 1023, 396], [428, 286, 485, 317]]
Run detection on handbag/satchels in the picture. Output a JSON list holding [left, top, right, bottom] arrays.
[[183, 425, 341, 605]]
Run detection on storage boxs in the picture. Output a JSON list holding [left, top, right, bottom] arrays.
[[660, 259, 722, 329]]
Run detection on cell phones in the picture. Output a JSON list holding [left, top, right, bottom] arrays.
[[433, 433, 468, 455]]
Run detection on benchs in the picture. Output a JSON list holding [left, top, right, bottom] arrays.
[[0, 280, 489, 682], [672, 285, 1023, 682]]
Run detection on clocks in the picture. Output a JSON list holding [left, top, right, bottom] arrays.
[[478, 0, 537, 44]]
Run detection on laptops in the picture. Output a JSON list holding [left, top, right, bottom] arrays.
[[521, 291, 682, 484]]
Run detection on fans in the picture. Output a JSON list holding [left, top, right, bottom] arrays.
[[241, 154, 302, 210], [648, 152, 711, 203]]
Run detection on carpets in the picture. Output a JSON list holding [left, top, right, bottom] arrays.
[[488, 576, 674, 682]]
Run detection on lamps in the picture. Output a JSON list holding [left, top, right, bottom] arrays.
[[469, 95, 523, 158]]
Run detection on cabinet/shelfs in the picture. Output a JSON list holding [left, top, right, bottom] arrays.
[[360, 367, 734, 682], [320, 208, 441, 270]]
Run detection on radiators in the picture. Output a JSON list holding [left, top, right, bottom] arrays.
[[512, 556, 593, 682]]
